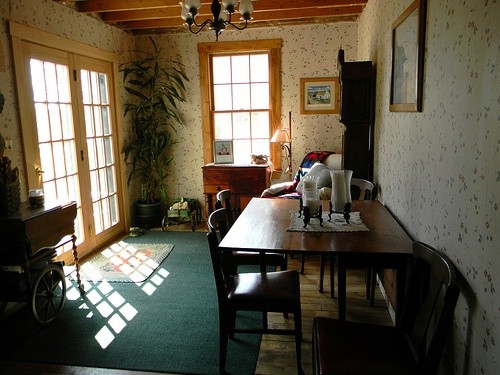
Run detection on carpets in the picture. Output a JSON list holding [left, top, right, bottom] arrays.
[[0, 230, 278, 375], [70, 241, 174, 283]]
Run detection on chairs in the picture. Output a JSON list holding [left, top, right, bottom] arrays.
[[215, 188, 287, 275], [259, 151, 344, 200], [312, 241, 456, 375], [206, 208, 305, 375], [300, 178, 375, 298]]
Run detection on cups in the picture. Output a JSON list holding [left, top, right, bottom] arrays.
[[302, 176, 321, 218], [330, 170, 353, 213]]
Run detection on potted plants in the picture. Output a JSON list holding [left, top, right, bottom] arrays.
[[117, 35, 190, 228]]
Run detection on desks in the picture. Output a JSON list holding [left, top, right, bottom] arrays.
[[217, 196, 414, 375], [0, 199, 84, 294]]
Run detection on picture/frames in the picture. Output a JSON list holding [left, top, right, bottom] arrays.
[[389, 0, 427, 112], [298, 77, 339, 115], [212, 140, 235, 164]]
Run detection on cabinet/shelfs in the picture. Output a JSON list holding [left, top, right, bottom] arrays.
[[336, 49, 374, 182], [201, 162, 272, 230]]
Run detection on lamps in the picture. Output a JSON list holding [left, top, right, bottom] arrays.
[[178, 0, 255, 43], [269, 110, 293, 180]]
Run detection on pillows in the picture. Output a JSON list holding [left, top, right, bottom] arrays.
[[295, 161, 332, 193]]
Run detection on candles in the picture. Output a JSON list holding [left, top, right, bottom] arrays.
[[331, 174, 346, 210], [304, 179, 320, 214]]
